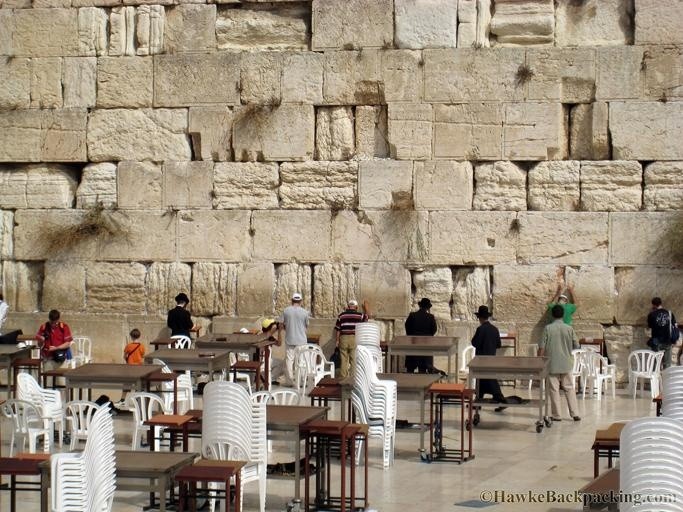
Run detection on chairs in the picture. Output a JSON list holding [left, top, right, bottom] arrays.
[[572, 347, 682, 512]]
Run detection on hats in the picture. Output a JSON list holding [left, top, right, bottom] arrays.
[[16, 342, 27, 349], [475, 306, 492, 317], [558, 295, 568, 304], [175, 293, 189, 304], [293, 293, 302, 300], [418, 298, 432, 307], [348, 300, 358, 306], [262, 319, 275, 329]]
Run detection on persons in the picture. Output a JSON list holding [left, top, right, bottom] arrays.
[[240, 292, 310, 387], [404, 297, 437, 374], [466, 305, 510, 413], [113, 328, 147, 408], [333, 298, 370, 378], [166, 291, 197, 374], [546, 283, 581, 326], [33, 308, 75, 390], [646, 297, 678, 371], [537, 304, 582, 422]]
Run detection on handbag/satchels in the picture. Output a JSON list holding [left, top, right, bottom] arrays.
[[669, 324, 679, 344], [54, 349, 65, 362], [647, 338, 659, 347], [330, 347, 341, 369]]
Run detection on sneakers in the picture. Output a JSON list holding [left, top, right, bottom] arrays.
[[114, 400, 126, 407], [466, 405, 507, 411], [550, 417, 580, 421], [259, 374, 295, 387]]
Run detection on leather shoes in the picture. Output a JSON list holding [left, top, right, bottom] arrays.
[[475, 396, 500, 403]]
